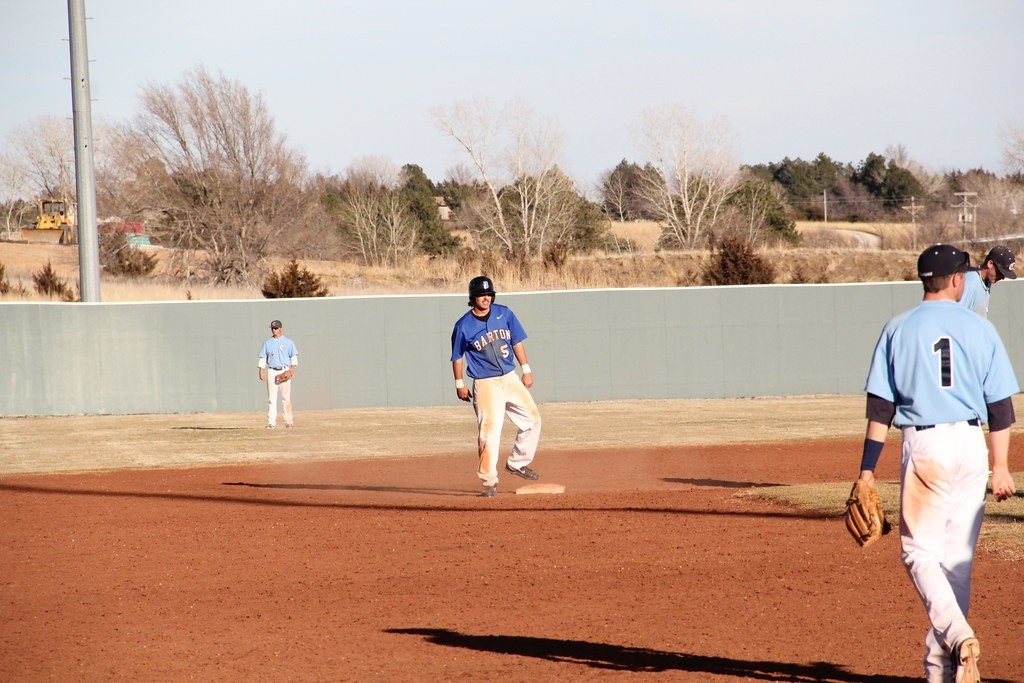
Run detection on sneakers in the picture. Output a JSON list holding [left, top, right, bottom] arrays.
[[506, 459, 539, 480], [481, 482, 496, 497], [950, 637, 982, 683]]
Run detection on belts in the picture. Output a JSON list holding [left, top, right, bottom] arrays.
[[270, 366, 285, 370], [914, 418, 979, 432]]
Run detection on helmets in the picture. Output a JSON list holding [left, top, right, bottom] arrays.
[[468, 276, 496, 306]]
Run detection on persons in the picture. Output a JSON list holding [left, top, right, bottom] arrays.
[[858, 245, 1019, 683], [958, 246, 1017, 318], [258, 320, 298, 429], [450, 277, 542, 496]]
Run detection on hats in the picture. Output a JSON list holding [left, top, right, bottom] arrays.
[[917, 244, 983, 279], [990, 245, 1017, 280], [271, 320, 282, 328]]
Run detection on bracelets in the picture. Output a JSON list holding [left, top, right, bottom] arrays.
[[454, 379, 464, 388], [522, 364, 531, 374]]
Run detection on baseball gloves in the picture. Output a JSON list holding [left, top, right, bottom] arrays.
[[274, 370, 292, 385], [844, 477, 892, 546]]
